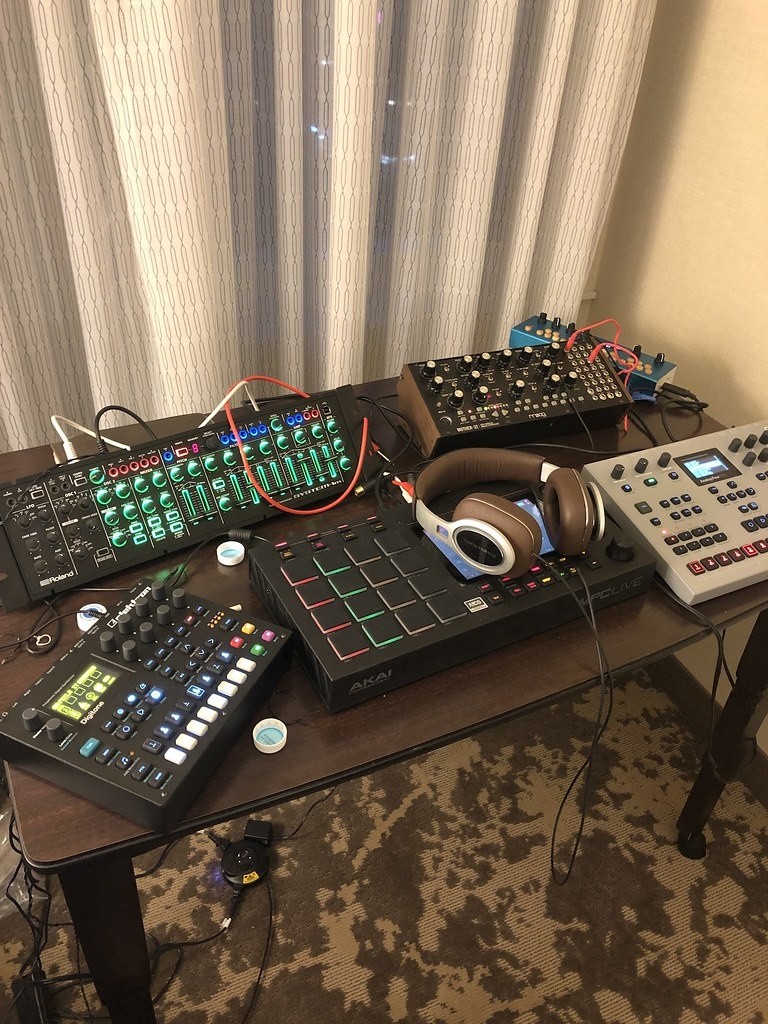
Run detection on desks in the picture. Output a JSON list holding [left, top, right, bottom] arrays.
[[2, 377, 767, 1024]]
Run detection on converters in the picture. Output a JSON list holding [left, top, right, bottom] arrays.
[[11, 970, 56, 1024]]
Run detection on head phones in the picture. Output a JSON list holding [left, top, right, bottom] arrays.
[[412, 448, 606, 579]]
